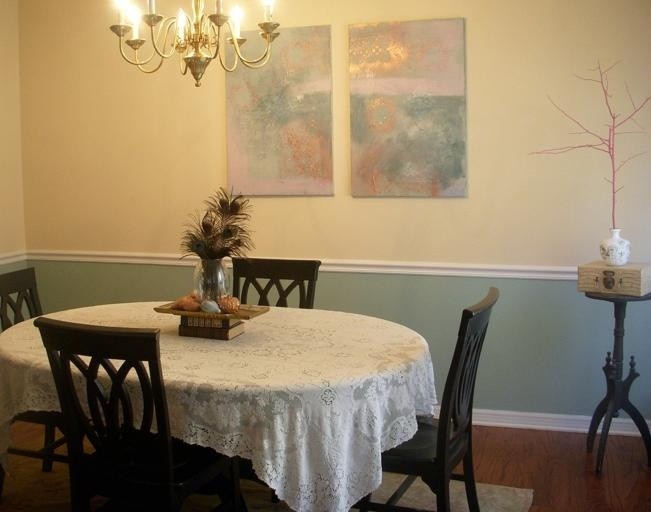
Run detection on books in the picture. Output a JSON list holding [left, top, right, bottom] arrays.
[[179, 315, 245, 341]]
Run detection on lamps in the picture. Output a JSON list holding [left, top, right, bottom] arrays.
[[109, 0, 281, 87]]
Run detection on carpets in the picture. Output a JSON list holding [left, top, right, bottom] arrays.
[[0, 421, 534, 512]]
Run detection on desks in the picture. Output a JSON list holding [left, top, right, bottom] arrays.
[[0, 301, 428, 512], [584, 293, 651, 479]]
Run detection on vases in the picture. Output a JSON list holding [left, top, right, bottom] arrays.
[[193, 257, 229, 302], [600, 229, 632, 266]]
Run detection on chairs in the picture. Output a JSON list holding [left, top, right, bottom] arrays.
[[233, 256, 321, 309], [33, 318, 247, 511], [352, 287, 499, 512], [0, 267, 98, 472]]
